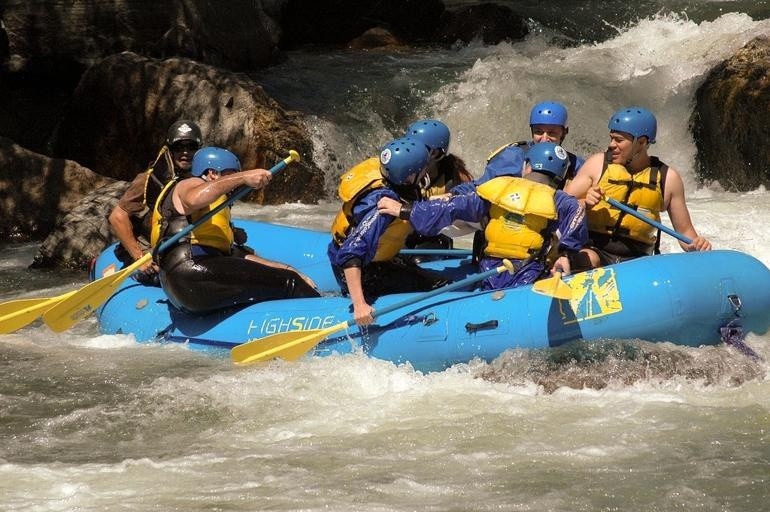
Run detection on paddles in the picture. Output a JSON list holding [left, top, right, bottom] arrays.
[[532, 267, 573, 300], [231, 258, 514, 368], [42, 150, 301, 334], [1, 269, 144, 334]]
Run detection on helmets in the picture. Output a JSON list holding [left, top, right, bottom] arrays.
[[166, 119, 203, 145], [406, 119, 450, 162], [524, 141, 571, 183], [607, 107, 657, 143], [190, 146, 242, 177], [529, 101, 568, 133], [378, 137, 430, 189]]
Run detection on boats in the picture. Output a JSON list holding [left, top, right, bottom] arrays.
[[86, 217, 770, 377]]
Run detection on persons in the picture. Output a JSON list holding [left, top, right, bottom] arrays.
[[327, 137, 452, 325], [108, 119, 255, 286], [151, 147, 322, 316], [381, 120, 471, 263], [377, 143, 589, 288], [564, 106, 711, 271], [429, 101, 585, 261]]
